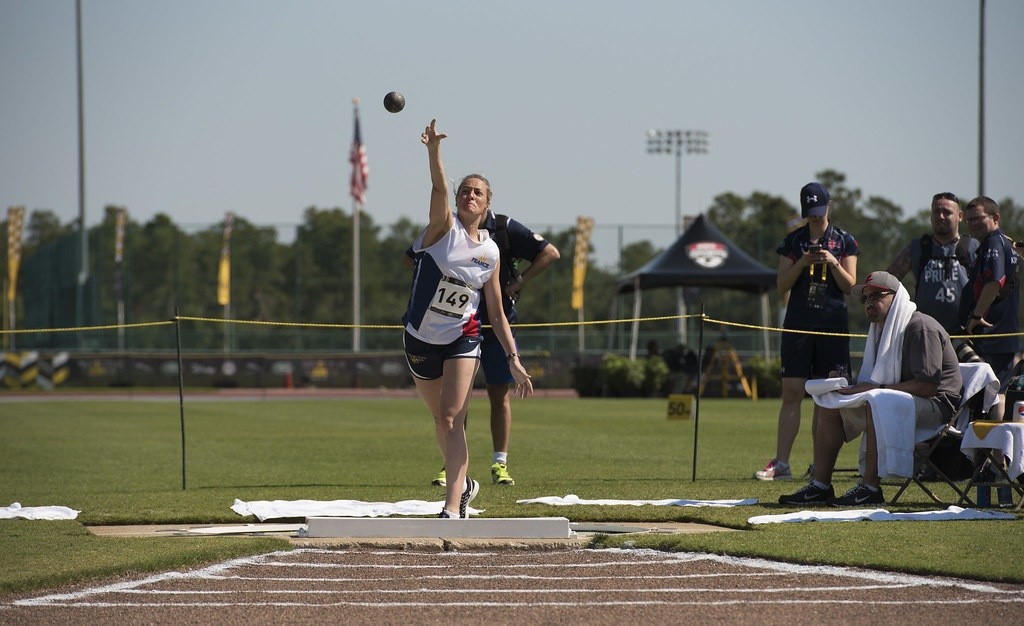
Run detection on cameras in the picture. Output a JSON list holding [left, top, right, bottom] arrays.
[[949, 326, 984, 363]]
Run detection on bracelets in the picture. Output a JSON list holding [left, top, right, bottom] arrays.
[[968, 309, 982, 320], [830, 262, 841, 270], [880, 384, 885, 388], [506, 353, 521, 362], [515, 274, 525, 286]]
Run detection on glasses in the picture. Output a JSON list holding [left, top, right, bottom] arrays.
[[965, 215, 989, 224], [933, 193, 960, 205], [859, 291, 896, 304]]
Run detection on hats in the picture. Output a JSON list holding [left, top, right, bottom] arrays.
[[800, 182, 829, 218], [850, 270, 900, 298]]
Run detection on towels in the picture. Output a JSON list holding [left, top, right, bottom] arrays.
[[751, 504, 1014, 526], [960, 422, 1024, 479], [958, 362, 1000, 413], [803, 377, 915, 478], [515, 493, 761, 508], [856, 282, 918, 385], [229, 496, 486, 524], [0, 501, 84, 521]]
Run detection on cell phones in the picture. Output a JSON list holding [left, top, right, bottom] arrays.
[[810, 245, 822, 254]]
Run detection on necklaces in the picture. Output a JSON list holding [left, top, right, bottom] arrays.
[[931, 233, 960, 256]]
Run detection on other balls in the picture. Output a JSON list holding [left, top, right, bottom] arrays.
[[383, 92, 406, 113]]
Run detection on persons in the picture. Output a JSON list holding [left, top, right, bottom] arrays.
[[778, 271, 963, 506], [885, 192, 1024, 392], [430, 207, 561, 488], [402, 119, 533, 521], [753, 183, 860, 481], [647, 341, 718, 379]]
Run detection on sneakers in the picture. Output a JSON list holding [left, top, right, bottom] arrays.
[[753, 459, 791, 481], [778, 483, 835, 506], [431, 466, 446, 487], [492, 463, 515, 486], [438, 475, 480, 519], [833, 483, 887, 508], [809, 464, 815, 480]]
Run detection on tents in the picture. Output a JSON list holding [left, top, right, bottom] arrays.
[[610, 213, 775, 361]]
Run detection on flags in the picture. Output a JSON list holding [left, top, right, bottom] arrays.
[[350, 109, 368, 204]]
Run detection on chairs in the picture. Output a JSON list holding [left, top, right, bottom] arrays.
[[860, 358, 1024, 512]]
